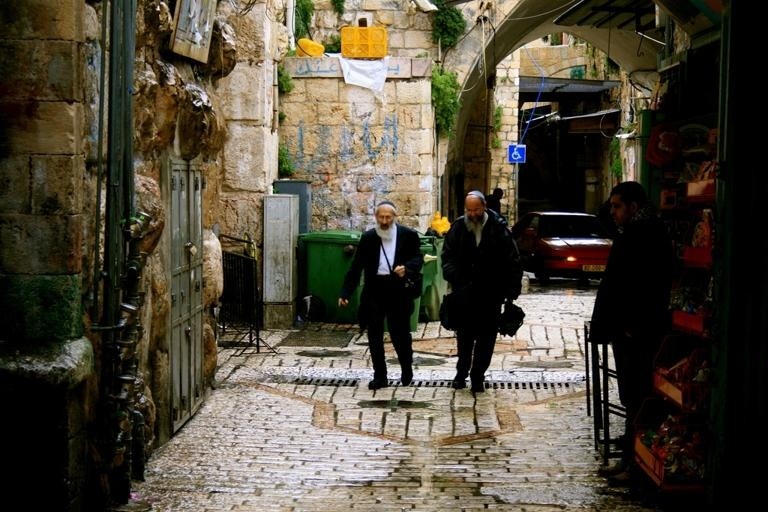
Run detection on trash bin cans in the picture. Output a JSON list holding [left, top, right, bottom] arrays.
[[297, 230, 449, 332]]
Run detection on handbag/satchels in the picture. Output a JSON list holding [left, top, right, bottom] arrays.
[[499, 301, 525, 338], [390, 272, 423, 299], [439, 289, 472, 330]]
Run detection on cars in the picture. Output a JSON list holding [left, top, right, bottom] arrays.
[[512, 209, 617, 292]]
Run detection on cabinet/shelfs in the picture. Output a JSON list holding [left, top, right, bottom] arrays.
[[632, 178, 716, 494]]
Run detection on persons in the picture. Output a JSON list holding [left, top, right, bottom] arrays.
[[589, 182, 673, 486], [338, 202, 424, 390], [441, 186, 523, 391]]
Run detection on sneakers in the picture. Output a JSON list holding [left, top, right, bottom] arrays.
[[401, 365, 413, 386], [452, 371, 484, 392], [598, 462, 632, 488], [369, 377, 388, 389]]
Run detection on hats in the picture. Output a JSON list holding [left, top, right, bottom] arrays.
[[374, 200, 397, 213], [468, 190, 485, 199]]
